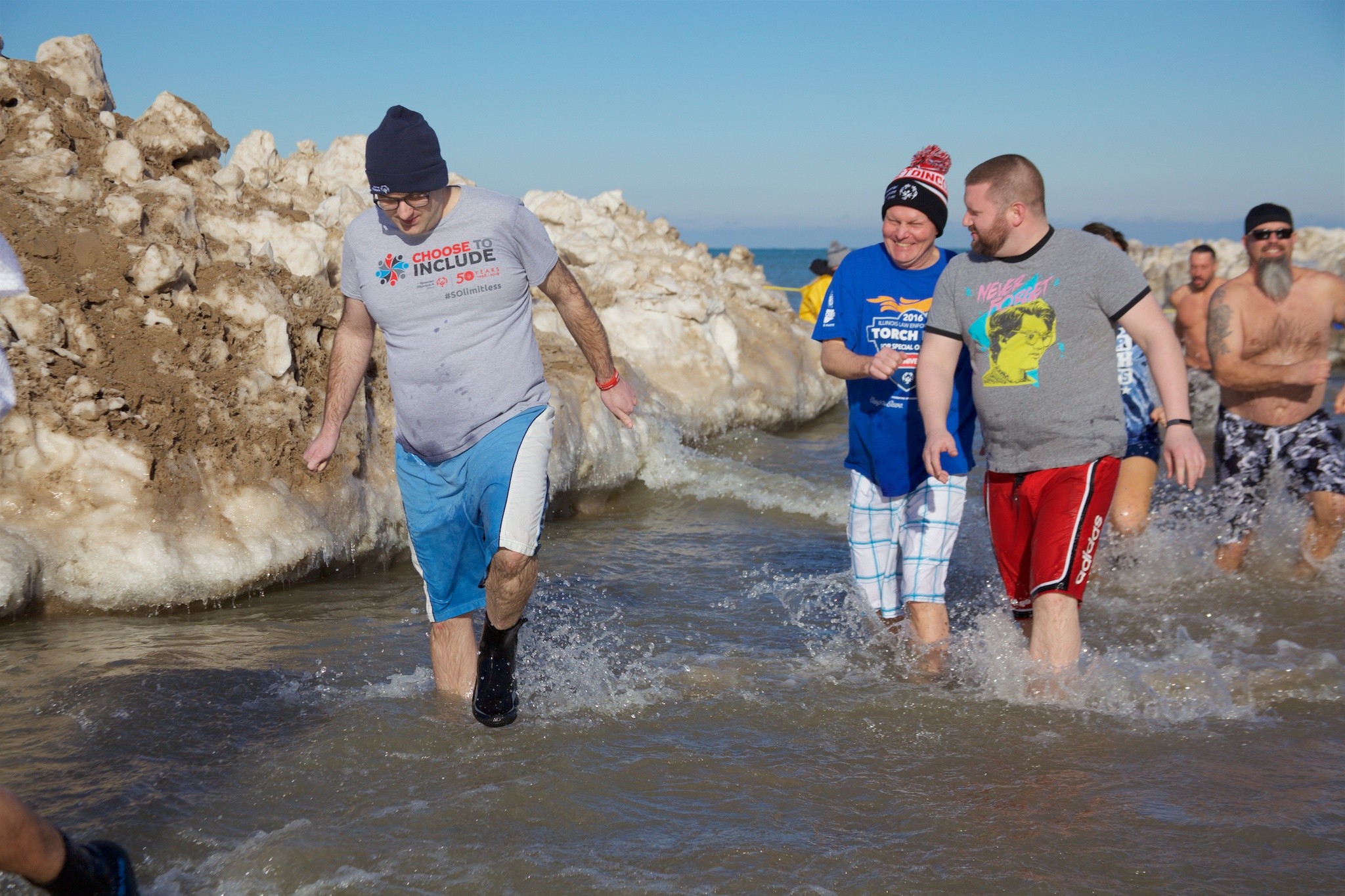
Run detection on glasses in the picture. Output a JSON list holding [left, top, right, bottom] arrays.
[[373, 190, 431, 211], [1248, 226, 1294, 240]]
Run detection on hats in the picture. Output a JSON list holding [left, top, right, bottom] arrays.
[[365, 105, 449, 198], [882, 145, 952, 242], [1245, 203, 1292, 236], [827, 241, 852, 269]]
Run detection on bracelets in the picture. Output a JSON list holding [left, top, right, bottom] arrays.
[[595, 367, 620, 390], [1166, 418, 1194, 429]]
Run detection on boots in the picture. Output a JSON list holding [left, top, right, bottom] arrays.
[[21, 830, 142, 896], [473, 610, 529, 728]]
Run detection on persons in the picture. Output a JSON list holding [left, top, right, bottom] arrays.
[[798, 241, 851, 325], [301, 106, 639, 730], [1205, 202, 1345, 572], [1078, 221, 1169, 536], [917, 154, 1207, 674], [0, 240, 141, 896], [813, 143, 979, 651], [1168, 245, 1228, 436]]
